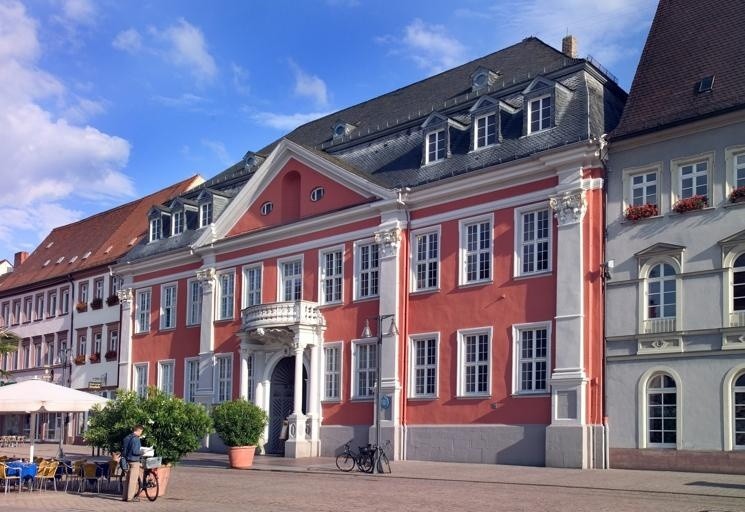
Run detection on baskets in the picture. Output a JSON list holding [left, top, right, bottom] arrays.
[[142, 457, 162, 469]]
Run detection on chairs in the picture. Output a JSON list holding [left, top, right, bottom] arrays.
[[0, 458, 123, 498]]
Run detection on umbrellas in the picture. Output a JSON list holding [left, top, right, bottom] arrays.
[[0, 379, 116, 463]]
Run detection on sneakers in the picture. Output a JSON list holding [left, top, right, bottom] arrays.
[[123, 498, 139, 502]]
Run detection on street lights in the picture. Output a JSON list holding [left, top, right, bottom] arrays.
[[361, 313, 400, 474]]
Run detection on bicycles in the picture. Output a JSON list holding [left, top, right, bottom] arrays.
[[117, 456, 163, 502], [336, 440, 392, 473], [55, 347, 74, 457]]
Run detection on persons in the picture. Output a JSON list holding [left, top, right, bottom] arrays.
[[122, 426, 148, 502]]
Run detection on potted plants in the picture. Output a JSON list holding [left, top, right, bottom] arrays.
[[83, 382, 269, 499]]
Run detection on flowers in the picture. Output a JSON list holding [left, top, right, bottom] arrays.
[[75, 350, 116, 365], [730, 186, 745, 202], [671, 195, 707, 213], [625, 203, 657, 221], [76, 295, 119, 311]]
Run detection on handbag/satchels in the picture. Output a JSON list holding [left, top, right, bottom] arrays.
[[119, 457, 128, 470]]
